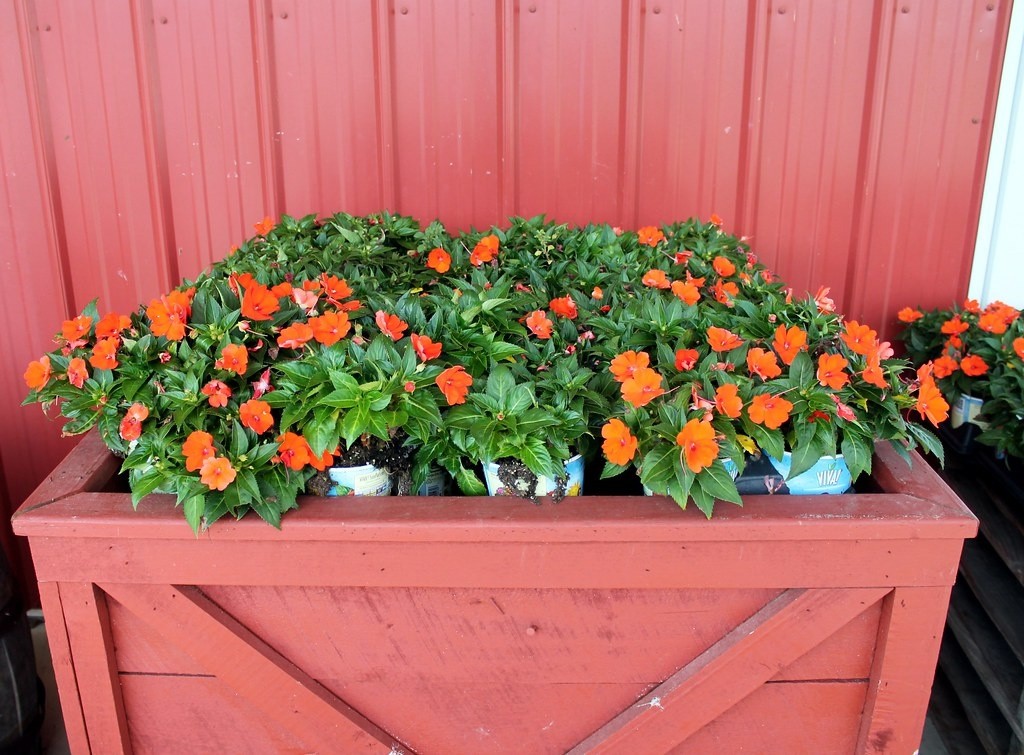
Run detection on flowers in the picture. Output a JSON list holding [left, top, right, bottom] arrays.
[[895, 297, 1024, 459], [21, 206, 949, 540]]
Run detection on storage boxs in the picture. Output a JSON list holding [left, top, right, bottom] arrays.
[[10, 427, 979, 755]]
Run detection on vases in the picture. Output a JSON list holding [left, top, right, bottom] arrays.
[[761, 448, 852, 495], [950, 393, 991, 432], [643, 457, 740, 496], [417, 467, 451, 497], [324, 465, 392, 496], [479, 454, 584, 496]]
